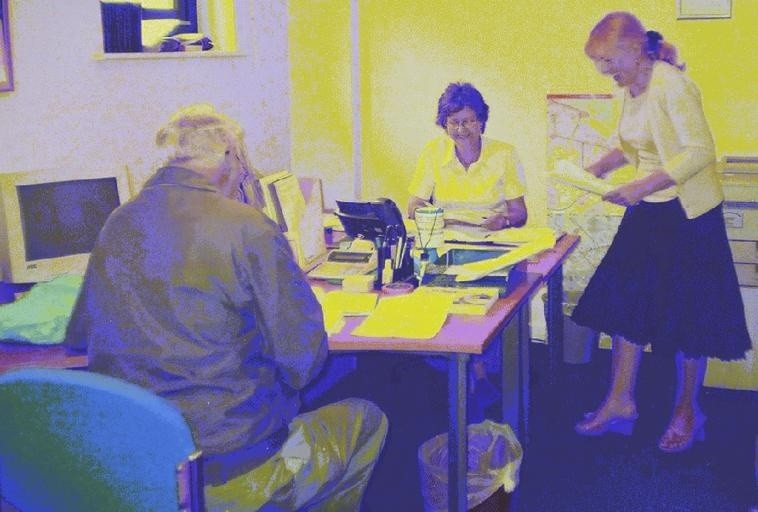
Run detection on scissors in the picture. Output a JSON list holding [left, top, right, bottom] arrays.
[[384, 224, 405, 246]]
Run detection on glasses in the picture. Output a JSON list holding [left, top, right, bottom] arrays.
[[226, 150, 250, 184], [443, 120, 481, 130]]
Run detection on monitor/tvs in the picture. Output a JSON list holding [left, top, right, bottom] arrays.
[[0, 165, 132, 284]]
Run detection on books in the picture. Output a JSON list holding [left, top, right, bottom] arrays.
[[252, 169, 291, 225], [411, 285, 502, 318], [267, 173, 309, 234], [324, 291, 380, 317]]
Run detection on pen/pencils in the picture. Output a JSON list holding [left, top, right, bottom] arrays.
[[375, 235, 409, 268], [482, 217, 490, 222]]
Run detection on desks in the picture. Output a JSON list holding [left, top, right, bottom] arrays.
[[305, 225, 581, 512]]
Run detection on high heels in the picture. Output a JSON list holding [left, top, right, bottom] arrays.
[[574, 407, 638, 438], [659, 410, 707, 453]]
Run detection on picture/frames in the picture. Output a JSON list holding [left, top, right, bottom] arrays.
[[677, 0, 731, 19], [0, 0, 15, 92]]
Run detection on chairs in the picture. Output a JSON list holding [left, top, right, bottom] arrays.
[[0, 367, 203, 512]]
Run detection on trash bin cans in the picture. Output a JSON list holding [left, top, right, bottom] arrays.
[[418, 426, 523, 512]]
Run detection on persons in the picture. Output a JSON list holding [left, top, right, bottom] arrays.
[[60, 102, 392, 512], [567, 10, 756, 460], [405, 80, 529, 415]]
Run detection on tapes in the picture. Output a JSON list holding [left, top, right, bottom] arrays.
[[381, 281, 415, 294]]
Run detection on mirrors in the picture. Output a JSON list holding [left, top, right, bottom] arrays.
[[100, 0, 247, 60]]
